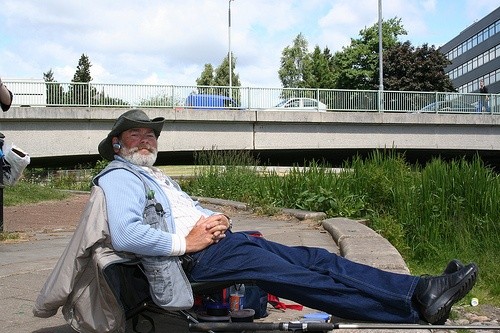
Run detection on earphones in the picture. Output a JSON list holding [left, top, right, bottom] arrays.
[[114, 144, 121, 149]]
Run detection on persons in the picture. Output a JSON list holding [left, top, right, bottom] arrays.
[[478, 80, 491, 114], [93, 108, 478, 325], [0, 77, 14, 236]]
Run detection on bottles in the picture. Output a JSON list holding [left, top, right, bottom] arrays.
[[230, 284, 245, 311]]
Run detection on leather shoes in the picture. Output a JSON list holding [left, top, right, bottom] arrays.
[[436, 259, 464, 325], [418, 263, 478, 323]]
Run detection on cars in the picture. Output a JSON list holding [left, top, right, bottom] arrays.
[[414, 100, 479, 114], [272, 97, 327, 113]]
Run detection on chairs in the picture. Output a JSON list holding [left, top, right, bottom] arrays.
[[31, 186, 267, 333]]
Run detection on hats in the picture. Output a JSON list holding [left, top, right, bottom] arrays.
[[98, 109, 165, 161]]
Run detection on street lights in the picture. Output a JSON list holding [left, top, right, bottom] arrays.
[[228, 0, 233, 90]]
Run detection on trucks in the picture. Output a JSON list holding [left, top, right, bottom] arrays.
[[187, 95, 243, 111], [1, 78, 47, 108]]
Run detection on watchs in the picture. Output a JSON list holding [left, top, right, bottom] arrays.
[[221, 212, 233, 234]]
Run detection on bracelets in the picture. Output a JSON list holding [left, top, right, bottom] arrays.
[[0, 82, 3, 89]]
